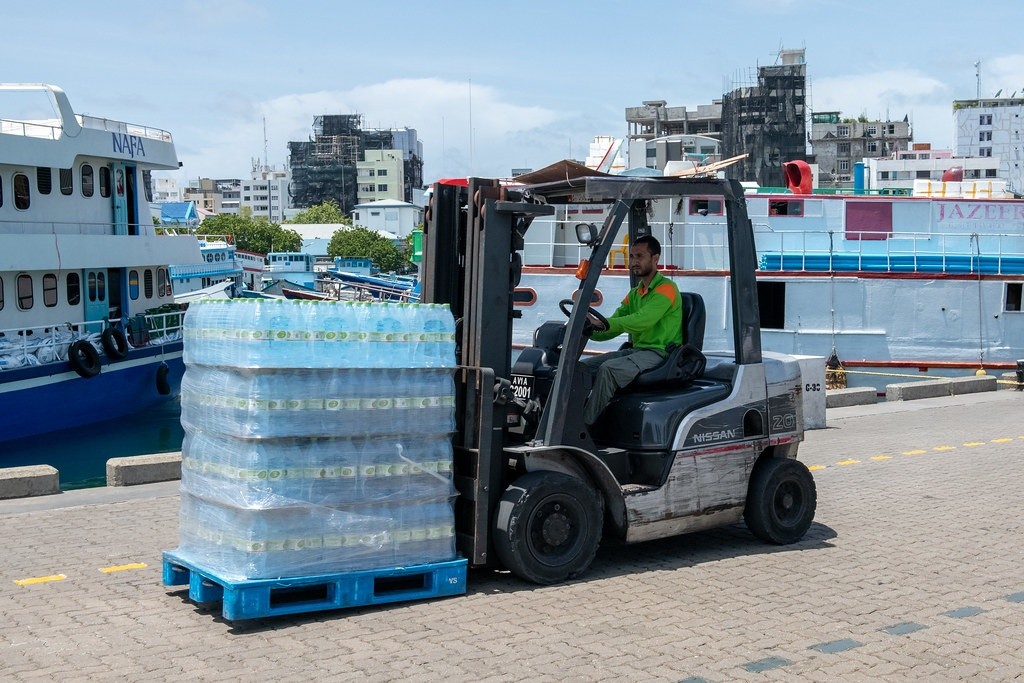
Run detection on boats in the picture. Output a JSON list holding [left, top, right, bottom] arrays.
[[282, 253, 420, 303], [0, 82, 245, 441], [327, 259, 416, 299]]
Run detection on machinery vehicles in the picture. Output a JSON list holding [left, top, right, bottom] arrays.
[[163, 159, 818, 621]]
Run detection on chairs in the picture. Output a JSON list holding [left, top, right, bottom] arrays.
[[617, 292, 706, 393]]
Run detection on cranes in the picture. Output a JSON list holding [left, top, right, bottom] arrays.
[[975, 60, 982, 99], [262, 115, 268, 172]]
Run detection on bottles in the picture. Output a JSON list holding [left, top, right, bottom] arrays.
[[179, 298, 458, 580]]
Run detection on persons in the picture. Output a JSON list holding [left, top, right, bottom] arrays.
[[580, 234, 683, 429]]
